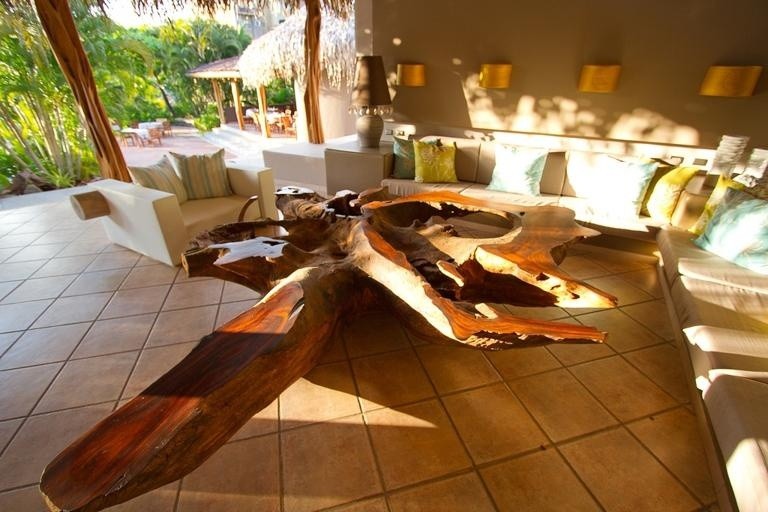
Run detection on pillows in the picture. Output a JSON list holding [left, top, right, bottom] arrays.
[[574, 150, 699, 232], [125, 148, 235, 205], [688, 174, 767, 277], [485, 141, 549, 198], [390, 135, 460, 185]]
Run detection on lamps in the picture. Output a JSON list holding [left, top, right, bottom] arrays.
[[478, 63, 512, 90], [696, 66, 761, 97], [577, 64, 621, 93], [394, 63, 427, 88], [347, 55, 393, 148]]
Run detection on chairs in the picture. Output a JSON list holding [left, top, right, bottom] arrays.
[[241, 108, 292, 135], [69, 153, 280, 267]]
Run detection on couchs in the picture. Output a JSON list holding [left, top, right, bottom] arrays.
[[381, 133, 708, 256], [655, 173, 767, 512]]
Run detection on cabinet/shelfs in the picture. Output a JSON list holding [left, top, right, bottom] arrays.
[[325, 140, 394, 197]]
